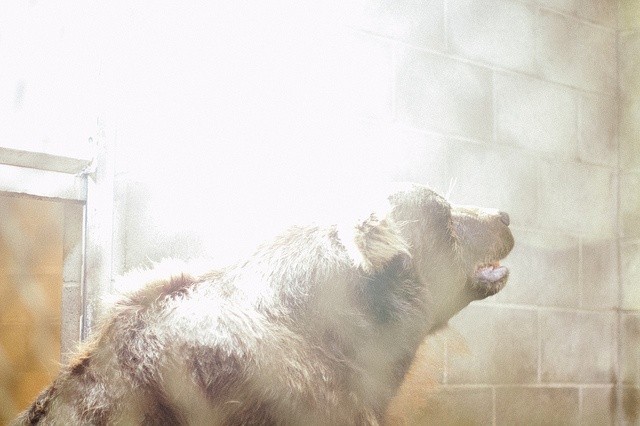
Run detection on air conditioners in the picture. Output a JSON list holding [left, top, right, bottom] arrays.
[[10, 185, 517, 426]]
